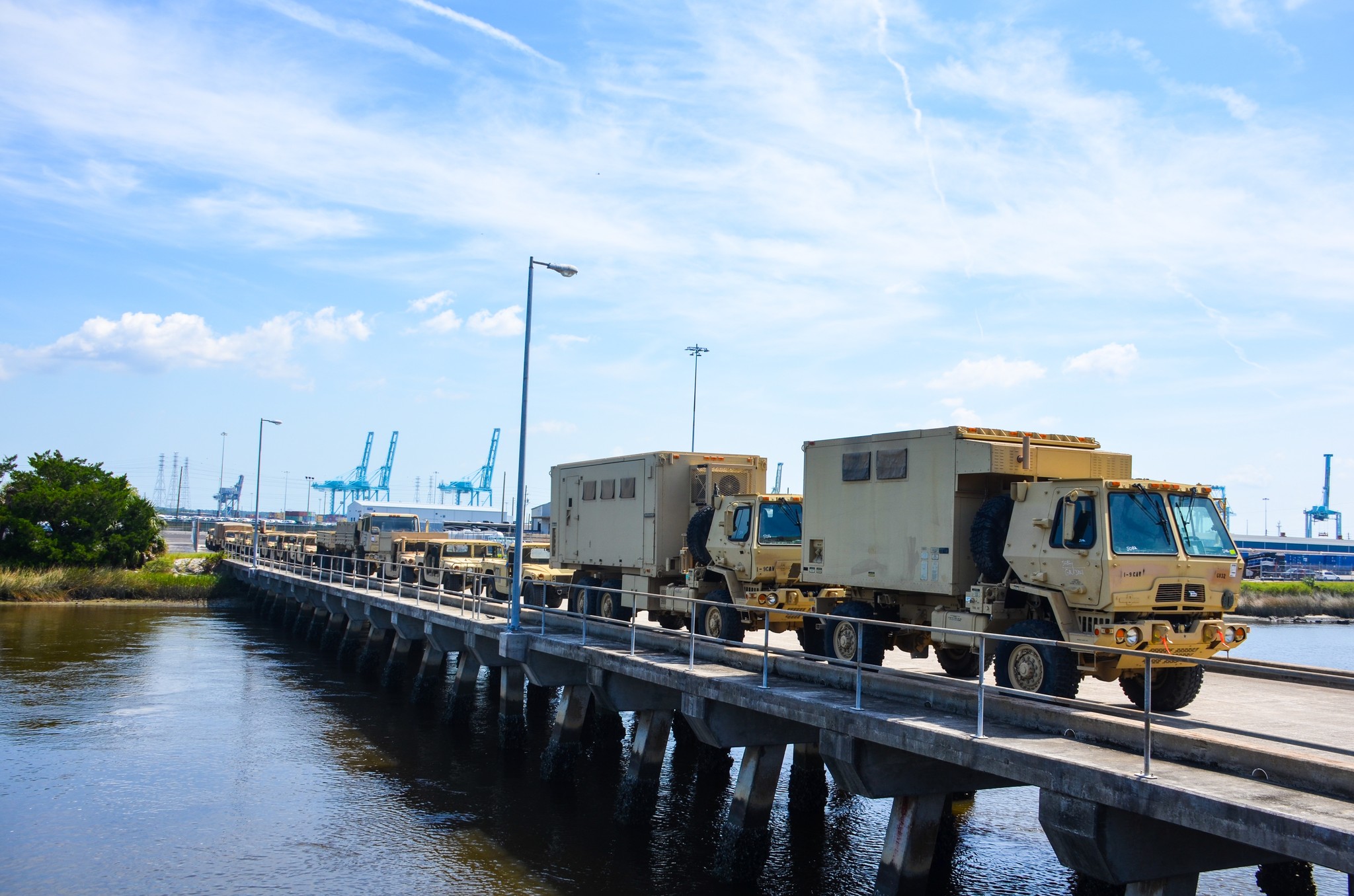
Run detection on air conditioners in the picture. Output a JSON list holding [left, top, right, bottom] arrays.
[[697, 471, 749, 502]]
